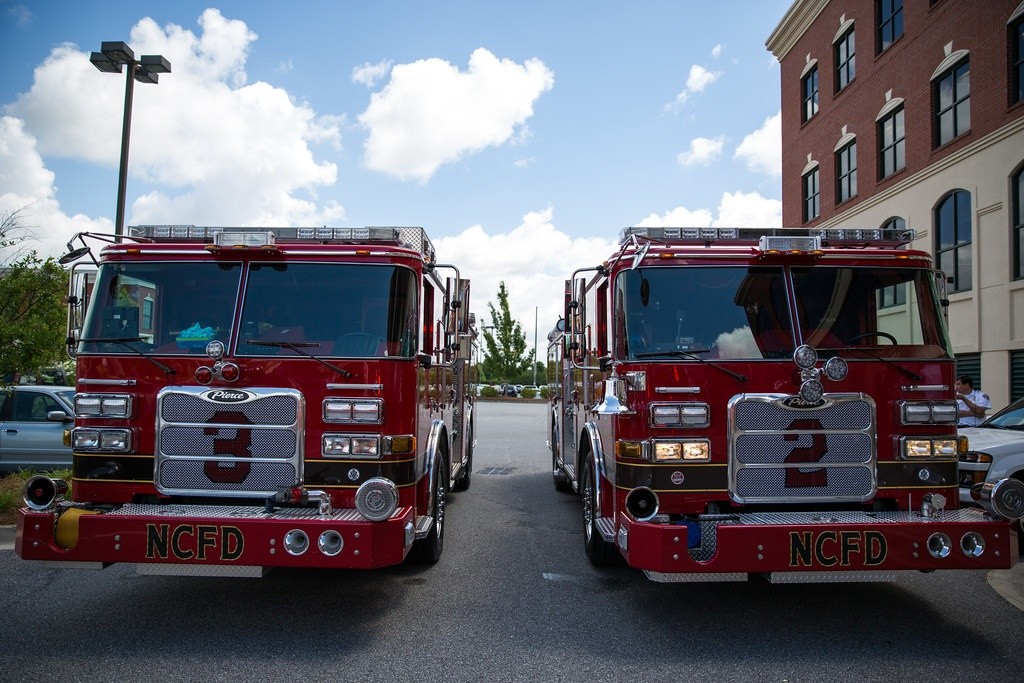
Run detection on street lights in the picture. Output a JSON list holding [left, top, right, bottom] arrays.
[[89, 42, 171, 245]]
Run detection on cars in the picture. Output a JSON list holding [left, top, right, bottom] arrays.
[[0, 382, 81, 481], [955, 398, 1023, 485], [15, 364, 70, 393]]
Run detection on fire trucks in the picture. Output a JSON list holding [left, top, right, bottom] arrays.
[[13, 224, 475, 572], [545, 225, 1014, 586]]
